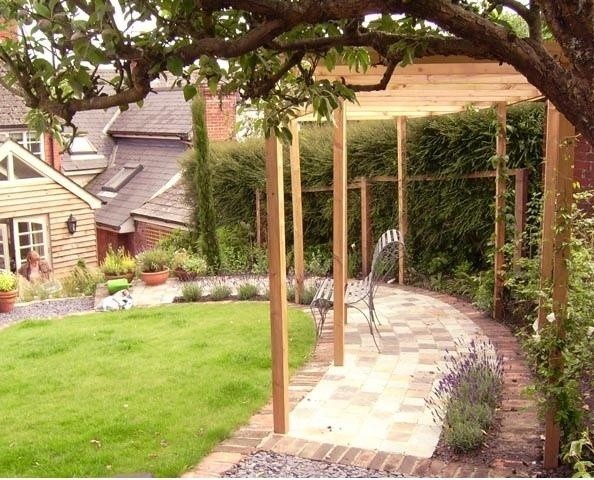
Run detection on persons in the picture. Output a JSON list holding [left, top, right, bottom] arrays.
[[18, 250, 52, 282]]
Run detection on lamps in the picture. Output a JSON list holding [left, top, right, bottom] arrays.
[[65, 213, 77, 234]]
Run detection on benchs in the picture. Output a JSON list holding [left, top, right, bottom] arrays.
[[310, 229, 406, 354]]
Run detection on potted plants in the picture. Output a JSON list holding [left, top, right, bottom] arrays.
[[101, 243, 207, 285], [0, 270, 18, 313]]
